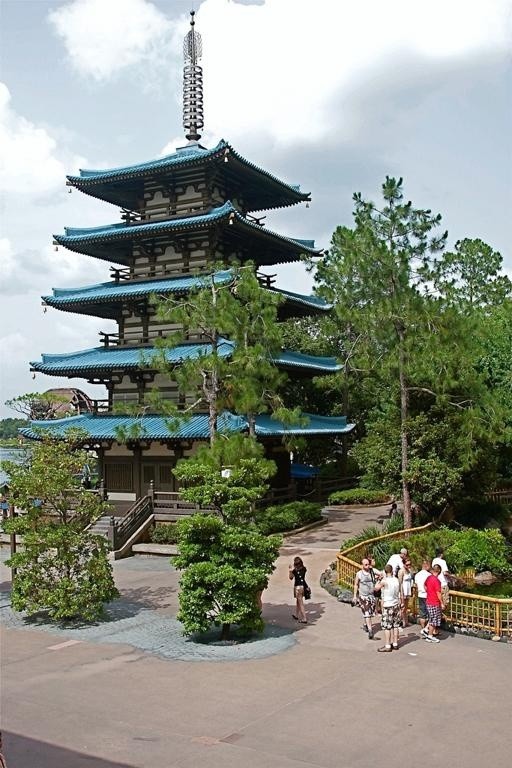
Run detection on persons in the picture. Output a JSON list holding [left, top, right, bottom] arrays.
[[257, 590, 266, 614], [0, 732, 7, 768], [390, 504, 397, 519], [352, 547, 459, 652], [288, 557, 307, 624]]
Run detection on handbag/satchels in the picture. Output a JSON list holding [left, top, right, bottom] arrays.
[[304, 587, 311, 600]]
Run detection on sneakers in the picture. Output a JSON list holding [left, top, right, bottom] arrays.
[[361, 624, 374, 639], [420, 628, 442, 643], [377, 642, 399, 652]]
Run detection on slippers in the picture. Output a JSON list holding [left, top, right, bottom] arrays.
[[291, 614, 307, 623]]
